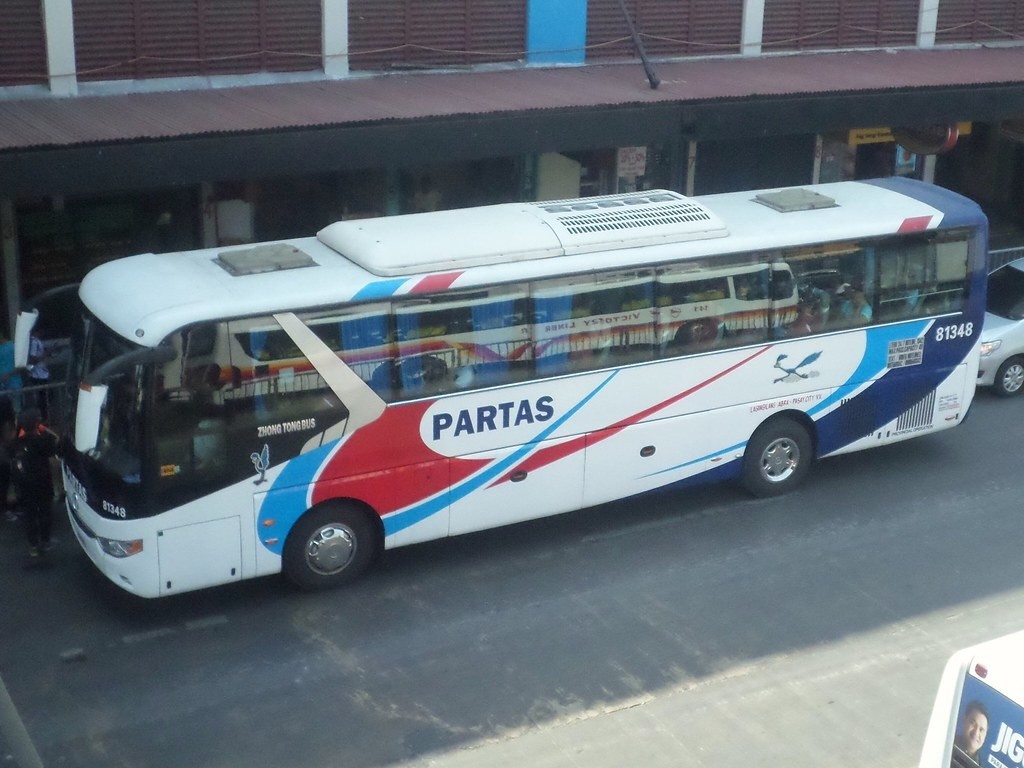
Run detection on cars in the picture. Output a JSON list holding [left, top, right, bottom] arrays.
[[971, 250, 1024, 401]]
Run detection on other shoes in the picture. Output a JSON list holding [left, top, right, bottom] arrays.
[[0, 511, 17, 522], [41, 539, 57, 552], [29, 546, 38, 556]]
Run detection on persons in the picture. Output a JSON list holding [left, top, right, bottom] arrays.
[[951, 701, 989, 768], [446, 350, 476, 393], [835, 284, 873, 327], [0, 325, 51, 437], [887, 265, 920, 317], [5, 407, 65, 557]]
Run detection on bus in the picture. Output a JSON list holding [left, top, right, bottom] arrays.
[[13, 177, 989, 593]]
